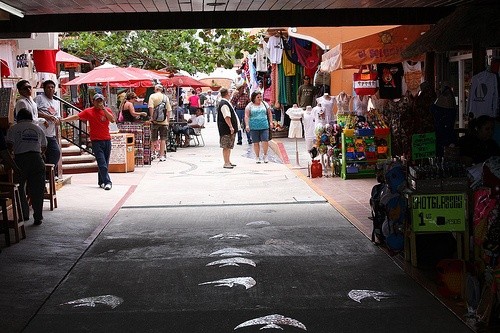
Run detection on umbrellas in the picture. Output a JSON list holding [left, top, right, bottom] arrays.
[[159, 75, 211, 122], [63, 62, 174, 109]]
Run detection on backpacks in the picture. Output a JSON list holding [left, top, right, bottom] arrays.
[[152, 94, 167, 122]]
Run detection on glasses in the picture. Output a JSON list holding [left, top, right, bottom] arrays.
[[304, 78, 311, 80], [20, 85, 32, 91]]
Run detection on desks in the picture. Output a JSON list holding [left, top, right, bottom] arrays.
[[170, 121, 188, 149]]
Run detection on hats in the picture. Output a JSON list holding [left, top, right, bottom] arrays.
[[126, 92, 137, 100], [207, 90, 211, 93], [236, 84, 245, 90], [93, 94, 104, 100], [154, 85, 163, 90]]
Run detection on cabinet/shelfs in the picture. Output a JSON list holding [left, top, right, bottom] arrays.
[[340, 132, 392, 180], [108, 133, 136, 174]]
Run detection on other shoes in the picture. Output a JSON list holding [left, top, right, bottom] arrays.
[[223, 166, 234, 169], [100, 183, 105, 188], [248, 142, 252, 144], [230, 163, 237, 166], [207, 120, 210, 122], [237, 142, 242, 145], [256, 157, 262, 164], [33, 219, 43, 226], [213, 120, 216, 122], [105, 185, 111, 190], [159, 157, 166, 161], [19, 217, 29, 221], [263, 155, 268, 163]]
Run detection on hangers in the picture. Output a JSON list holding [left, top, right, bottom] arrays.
[[273, 30, 280, 37]]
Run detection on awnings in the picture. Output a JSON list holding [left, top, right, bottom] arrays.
[[320, 24, 433, 72]]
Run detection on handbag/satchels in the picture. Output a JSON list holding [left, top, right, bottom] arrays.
[[269, 129, 272, 141], [118, 104, 124, 122]]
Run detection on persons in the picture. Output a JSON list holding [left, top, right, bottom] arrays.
[[297, 75, 318, 138], [316, 90, 334, 124], [188, 88, 209, 119], [285, 104, 326, 142], [59, 94, 115, 190], [335, 91, 352, 115], [217, 89, 241, 168], [230, 84, 252, 145], [173, 108, 205, 148], [245, 90, 274, 164], [256, 81, 265, 101], [6, 108, 47, 225], [352, 93, 369, 112], [122, 92, 147, 124], [14, 79, 61, 182], [371, 89, 394, 112], [205, 91, 216, 122], [147, 84, 172, 162]]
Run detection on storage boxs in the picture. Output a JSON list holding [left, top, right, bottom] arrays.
[[407, 175, 470, 194], [342, 126, 391, 174]]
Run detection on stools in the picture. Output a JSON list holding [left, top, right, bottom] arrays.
[[0, 198, 11, 254], [0, 182, 26, 244], [29, 164, 57, 211]]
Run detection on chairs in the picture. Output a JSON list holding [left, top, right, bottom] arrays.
[[189, 127, 205, 147]]
